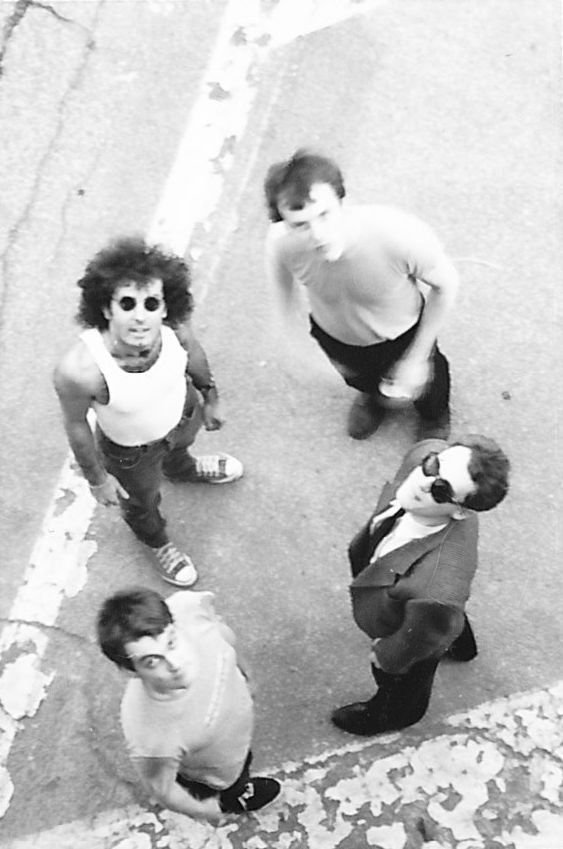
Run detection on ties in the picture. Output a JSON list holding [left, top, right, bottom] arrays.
[[368, 508, 405, 561]]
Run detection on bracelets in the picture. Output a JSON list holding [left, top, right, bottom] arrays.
[[85, 473, 112, 489], [191, 371, 217, 393]]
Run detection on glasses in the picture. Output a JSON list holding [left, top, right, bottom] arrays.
[[112, 296, 163, 311], [422, 452, 464, 506]]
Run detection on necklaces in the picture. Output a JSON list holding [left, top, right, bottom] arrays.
[[108, 329, 163, 372]]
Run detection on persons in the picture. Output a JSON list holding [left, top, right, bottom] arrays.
[[330, 433, 512, 736], [52, 233, 244, 588], [263, 148, 459, 444], [97, 586, 284, 819]]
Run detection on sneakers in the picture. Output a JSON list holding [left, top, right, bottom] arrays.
[[220, 777, 283, 813], [161, 453, 242, 484], [141, 540, 197, 587]]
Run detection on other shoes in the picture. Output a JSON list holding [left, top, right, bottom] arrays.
[[418, 406, 450, 441], [348, 395, 382, 440], [330, 702, 404, 736]]
